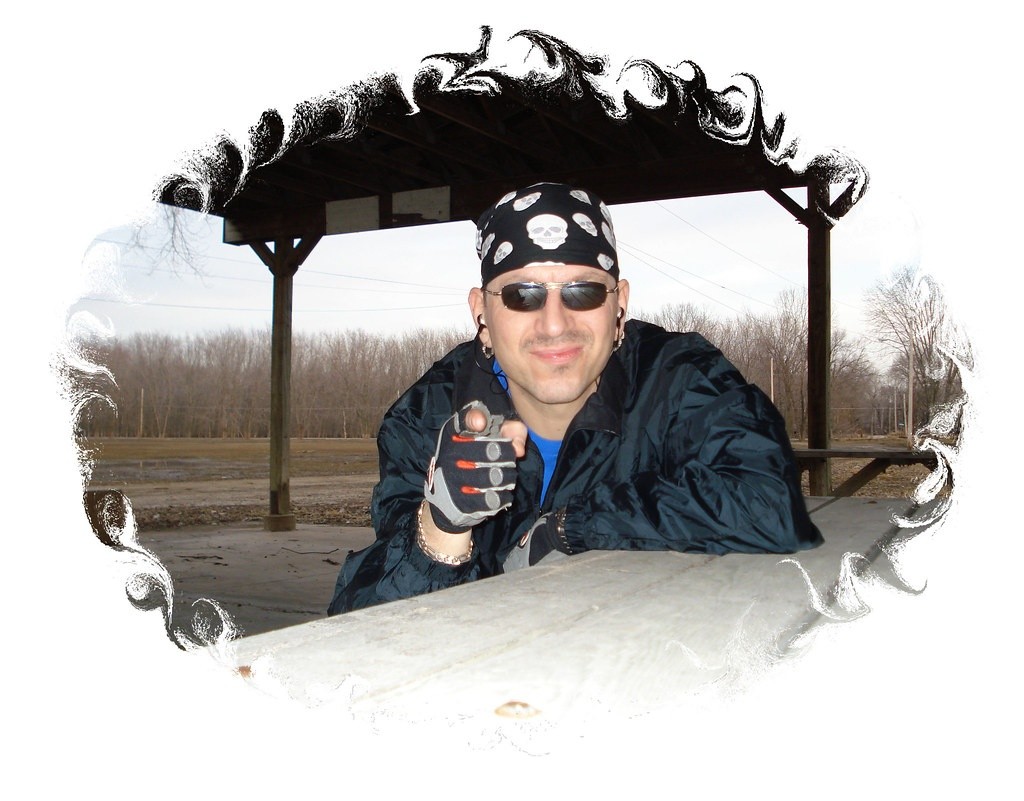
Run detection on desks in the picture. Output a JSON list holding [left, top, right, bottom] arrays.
[[197, 492, 934, 665]]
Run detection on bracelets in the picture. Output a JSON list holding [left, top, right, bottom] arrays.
[[415, 499, 474, 566], [555, 506, 572, 555]]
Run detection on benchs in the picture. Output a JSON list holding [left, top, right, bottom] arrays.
[[784, 441, 957, 501]]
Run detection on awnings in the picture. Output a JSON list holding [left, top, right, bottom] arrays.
[[157, 31, 870, 526]]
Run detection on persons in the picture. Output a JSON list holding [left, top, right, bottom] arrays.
[[328, 183, 823, 611]]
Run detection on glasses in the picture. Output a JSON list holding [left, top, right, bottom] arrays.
[[483, 280, 619, 312]]
